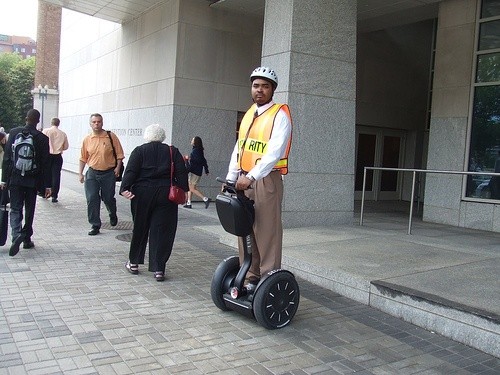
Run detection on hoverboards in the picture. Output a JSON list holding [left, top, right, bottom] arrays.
[[211, 175, 300, 330]]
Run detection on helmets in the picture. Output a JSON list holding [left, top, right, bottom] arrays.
[[250, 67, 278, 90]]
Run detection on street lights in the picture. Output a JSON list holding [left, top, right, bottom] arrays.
[[37, 84, 48, 133]]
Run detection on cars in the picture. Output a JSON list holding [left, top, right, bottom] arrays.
[[473, 179, 490, 199]]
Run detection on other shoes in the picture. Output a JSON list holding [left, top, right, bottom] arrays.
[[23, 240, 34, 248], [88, 225, 102, 235], [110, 208, 117, 227], [9, 232, 27, 256], [204, 198, 211, 209], [183, 204, 192, 208], [52, 198, 58, 203]]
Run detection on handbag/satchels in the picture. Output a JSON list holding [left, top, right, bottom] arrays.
[[216, 187, 256, 237], [0, 188, 8, 247], [113, 162, 125, 182], [169, 185, 187, 206]]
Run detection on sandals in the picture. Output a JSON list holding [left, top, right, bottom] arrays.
[[125, 260, 138, 273], [153, 271, 166, 281]]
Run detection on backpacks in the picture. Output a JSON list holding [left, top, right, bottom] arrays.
[[13, 129, 44, 176]]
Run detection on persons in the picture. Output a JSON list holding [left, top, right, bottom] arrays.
[[77, 113, 125, 234], [118, 123, 189, 281], [182, 135, 212, 210], [222, 65, 292, 290], [1, 108, 53, 255], [0, 131, 11, 247], [41, 118, 69, 203]]
